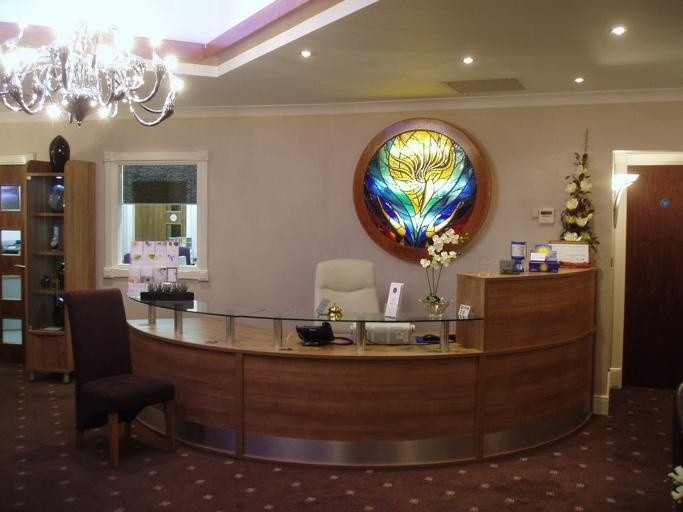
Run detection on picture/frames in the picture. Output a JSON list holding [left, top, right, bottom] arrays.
[[354, 116, 497, 265], [2, 319, 22, 345], [2, 230, 20, 256], [1, 274, 21, 300], [0, 185, 21, 212]]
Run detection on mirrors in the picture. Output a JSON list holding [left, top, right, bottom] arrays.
[[104, 150, 207, 282]]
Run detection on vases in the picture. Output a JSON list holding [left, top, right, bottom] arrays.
[[424, 295, 452, 319]]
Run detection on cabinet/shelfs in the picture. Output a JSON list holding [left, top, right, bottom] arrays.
[[24, 161, 94, 383]]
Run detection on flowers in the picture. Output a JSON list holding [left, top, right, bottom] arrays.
[[421, 230, 470, 299], [560, 130, 601, 265]]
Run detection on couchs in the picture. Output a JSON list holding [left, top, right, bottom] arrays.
[[315, 259, 381, 335]]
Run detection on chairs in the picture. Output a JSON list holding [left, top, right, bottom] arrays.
[[63, 288, 176, 464]]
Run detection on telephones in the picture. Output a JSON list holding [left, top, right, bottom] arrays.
[[295, 322, 353, 346]]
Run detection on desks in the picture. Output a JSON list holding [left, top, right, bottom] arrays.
[[128, 268, 598, 470]]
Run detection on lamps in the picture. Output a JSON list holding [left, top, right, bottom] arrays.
[[612, 170, 641, 228], [0, 10, 187, 127]]
